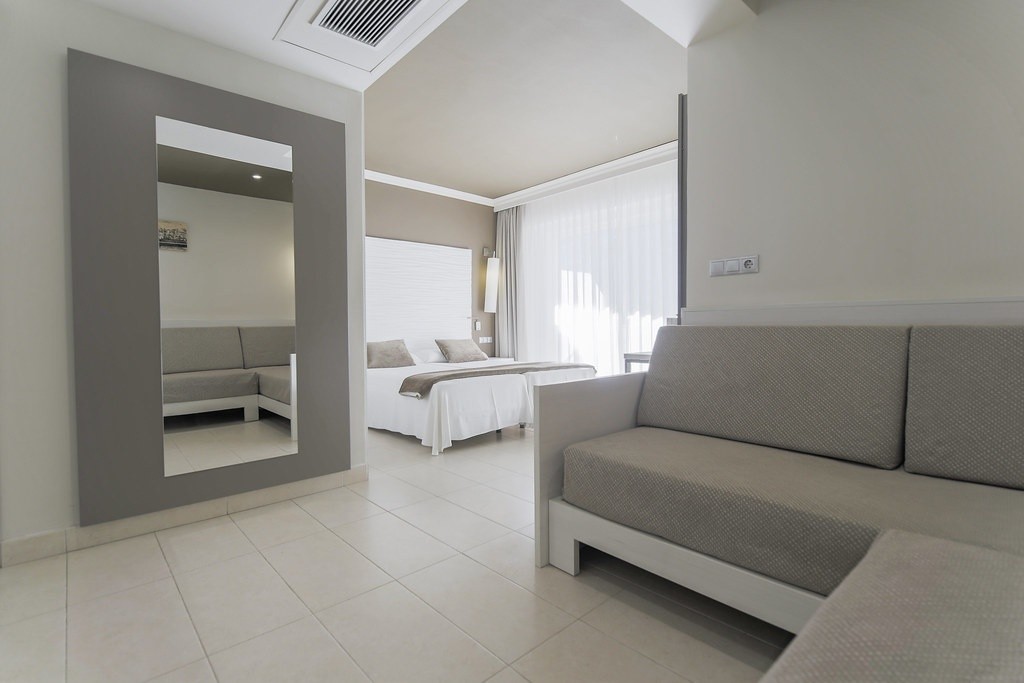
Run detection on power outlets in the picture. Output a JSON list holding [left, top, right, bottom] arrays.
[[476, 322, 481, 330], [484, 337, 488, 343], [741, 255, 759, 273], [489, 337, 493, 343]]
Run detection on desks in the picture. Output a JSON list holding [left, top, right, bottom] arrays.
[[623, 353, 654, 372]]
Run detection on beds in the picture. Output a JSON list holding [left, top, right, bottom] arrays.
[[365, 338, 597, 455]]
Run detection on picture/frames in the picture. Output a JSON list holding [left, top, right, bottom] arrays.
[[159, 221, 190, 254]]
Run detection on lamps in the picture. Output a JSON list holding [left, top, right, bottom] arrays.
[[482, 248, 499, 313]]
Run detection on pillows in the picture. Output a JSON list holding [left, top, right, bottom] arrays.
[[636, 324, 910, 470], [366, 339, 416, 369], [161, 327, 243, 373], [240, 325, 296, 370], [435, 339, 489, 364], [903, 327, 1024, 491]]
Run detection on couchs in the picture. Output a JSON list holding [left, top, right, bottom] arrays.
[[161, 324, 296, 438], [530, 324, 1024, 683]]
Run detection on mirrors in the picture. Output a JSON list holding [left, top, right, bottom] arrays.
[[155, 118, 296, 477]]
